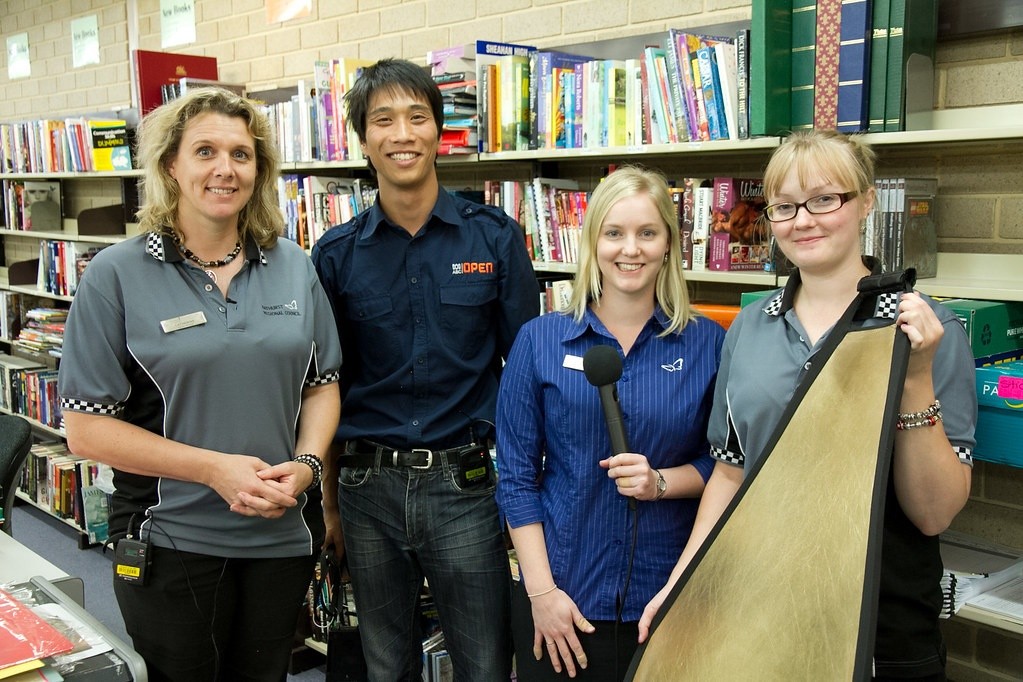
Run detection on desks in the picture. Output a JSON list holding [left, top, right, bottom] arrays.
[[0, 529, 85, 609]]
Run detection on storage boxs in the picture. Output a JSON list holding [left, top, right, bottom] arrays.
[[931, 294, 1023, 469], [691, 288, 781, 331]]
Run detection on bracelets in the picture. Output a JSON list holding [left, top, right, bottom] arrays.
[[896, 400, 942, 430], [528, 585, 557, 598], [293, 454, 324, 492]]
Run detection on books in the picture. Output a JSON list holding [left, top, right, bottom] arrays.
[[0, 117, 132, 544], [163, 0, 939, 316], [305, 563, 359, 643], [419, 594, 453, 682]]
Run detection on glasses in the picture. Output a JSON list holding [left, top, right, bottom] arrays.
[[763, 190, 863, 222]]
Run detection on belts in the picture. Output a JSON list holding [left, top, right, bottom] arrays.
[[358, 441, 485, 470]]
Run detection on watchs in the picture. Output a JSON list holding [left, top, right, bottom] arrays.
[[652, 469, 667, 501]]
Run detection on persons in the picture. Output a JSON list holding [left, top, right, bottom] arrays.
[[637, 131, 975, 682], [60, 88, 343, 682], [312, 59, 541, 682], [24, 190, 52, 216], [495, 167, 728, 682]]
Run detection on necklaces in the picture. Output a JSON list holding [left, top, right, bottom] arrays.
[[172, 231, 242, 267]]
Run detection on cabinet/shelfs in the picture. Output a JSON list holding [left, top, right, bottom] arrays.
[[0, 168, 147, 552], [0, 575, 147, 682], [275, 122, 1023, 304], [939, 542, 1023, 682]]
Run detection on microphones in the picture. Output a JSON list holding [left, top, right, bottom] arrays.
[[583, 344, 636, 511]]
